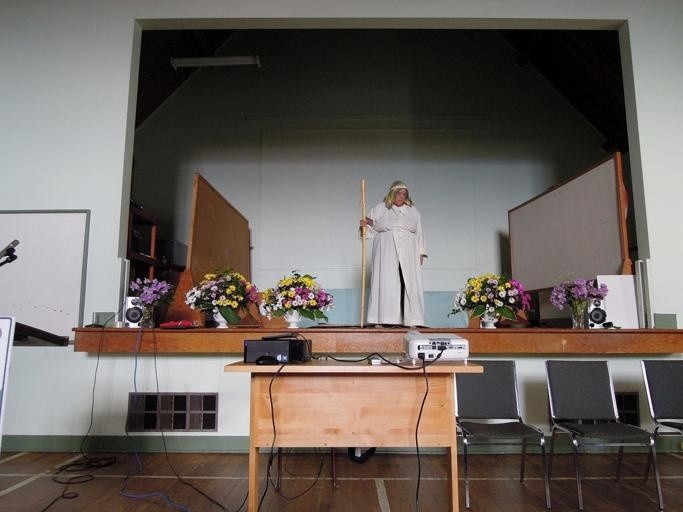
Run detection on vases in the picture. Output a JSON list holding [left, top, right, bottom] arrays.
[[284, 306, 301, 328], [140, 305, 155, 329], [480, 311, 501, 329], [570, 299, 589, 330], [211, 304, 229, 329]]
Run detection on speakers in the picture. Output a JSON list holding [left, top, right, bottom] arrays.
[[583, 294, 608, 330], [124, 295, 152, 327]]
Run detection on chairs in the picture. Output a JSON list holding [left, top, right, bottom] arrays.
[[640, 359, 683, 509], [453, 359, 551, 512], [544, 359, 667, 512]]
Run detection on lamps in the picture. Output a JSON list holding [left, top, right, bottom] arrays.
[[169, 54, 261, 69]]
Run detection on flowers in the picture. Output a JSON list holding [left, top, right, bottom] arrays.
[[255, 273, 336, 323], [549, 277, 609, 310], [129, 277, 174, 310], [184, 271, 261, 310], [448, 272, 533, 320]]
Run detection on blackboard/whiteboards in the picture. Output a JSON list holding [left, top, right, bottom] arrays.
[[508, 151, 629, 294], [0, 209, 90, 346]]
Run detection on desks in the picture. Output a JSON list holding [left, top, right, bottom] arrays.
[[225, 352, 484, 512]]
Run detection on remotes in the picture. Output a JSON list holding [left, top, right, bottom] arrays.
[[261, 331, 298, 339]]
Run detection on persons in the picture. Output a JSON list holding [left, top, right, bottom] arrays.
[[357, 181, 428, 329]]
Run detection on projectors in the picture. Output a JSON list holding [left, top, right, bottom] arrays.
[[244, 339, 312, 363], [402, 332, 470, 366]]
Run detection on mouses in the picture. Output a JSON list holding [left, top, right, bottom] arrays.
[[256, 355, 278, 365]]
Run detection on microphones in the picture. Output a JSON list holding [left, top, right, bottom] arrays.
[[0, 239, 20, 261]]
